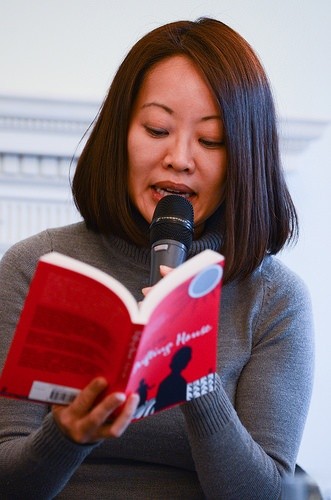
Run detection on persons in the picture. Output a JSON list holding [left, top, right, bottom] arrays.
[[0, 17, 326, 500]]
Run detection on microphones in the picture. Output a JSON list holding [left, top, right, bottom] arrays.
[[148, 194, 194, 287]]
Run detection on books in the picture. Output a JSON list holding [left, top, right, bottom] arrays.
[[1, 247, 224, 431]]
